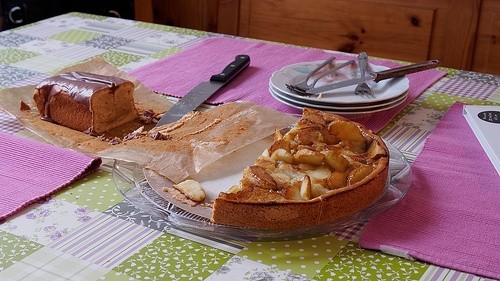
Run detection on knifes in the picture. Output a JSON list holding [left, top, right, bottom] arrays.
[[307, 60, 438, 93], [157, 55, 250, 128]]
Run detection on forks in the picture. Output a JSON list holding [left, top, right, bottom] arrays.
[[288, 51, 372, 95]]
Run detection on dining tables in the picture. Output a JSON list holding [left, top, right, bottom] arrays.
[[1, 12, 500, 281]]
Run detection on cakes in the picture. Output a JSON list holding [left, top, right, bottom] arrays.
[[212, 108, 390, 227], [172, 179, 206, 202]]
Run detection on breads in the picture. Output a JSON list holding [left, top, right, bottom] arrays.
[[33, 71, 141, 135]]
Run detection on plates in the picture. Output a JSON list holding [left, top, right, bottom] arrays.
[[113, 138, 412, 243], [269, 62, 408, 114]]
